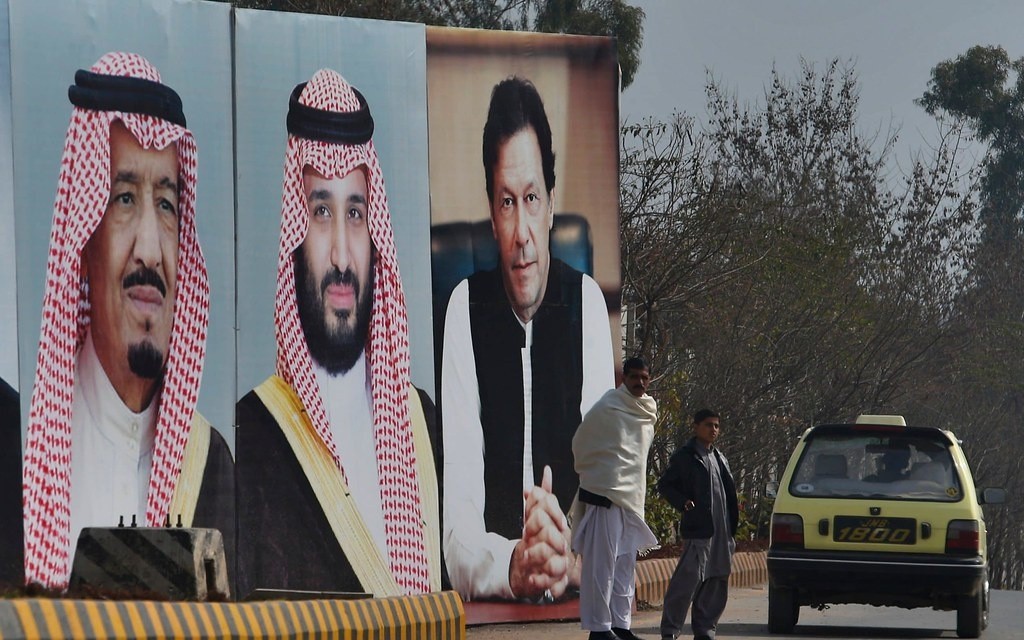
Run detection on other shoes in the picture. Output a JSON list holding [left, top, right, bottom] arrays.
[[611, 627, 645, 640], [589, 630, 622, 640], [694, 635, 713, 640], [662, 634, 677, 640]]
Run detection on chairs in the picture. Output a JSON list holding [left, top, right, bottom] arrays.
[[811, 454, 850, 480], [911, 462, 947, 484]]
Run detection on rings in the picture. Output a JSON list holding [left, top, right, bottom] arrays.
[[543, 589, 554, 602]]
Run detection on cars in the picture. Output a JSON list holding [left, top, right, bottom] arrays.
[[763, 413, 1012, 637]]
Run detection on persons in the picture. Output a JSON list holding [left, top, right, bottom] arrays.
[[861, 440, 911, 483], [572, 357, 657, 640], [23, 50, 236, 599], [657, 409, 739, 640], [442, 77, 617, 605], [235, 68, 442, 602]]
